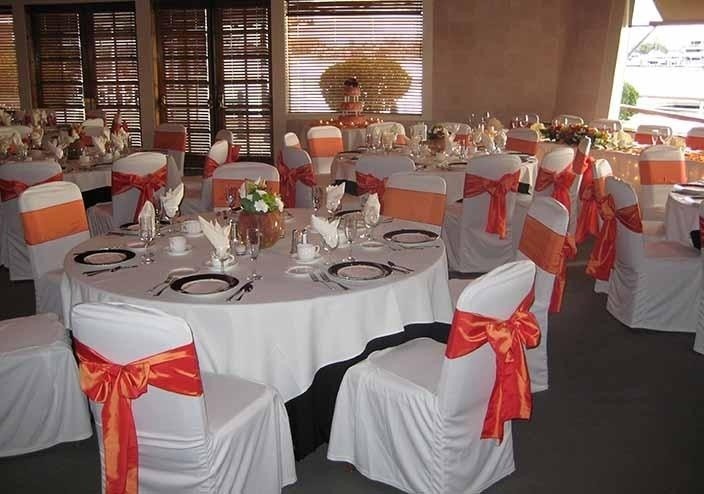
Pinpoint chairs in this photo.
[71,301,284,493]
[0,312,94,460]
[17,180,91,323]
[344,258,536,494]
[212,162,281,212]
[280,119,592,272]
[448,194,572,394]
[383,173,448,227]
[1,116,187,279]
[493,117,703,239]
[591,144,703,356]
[179,129,240,214]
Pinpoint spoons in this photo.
[380,260,416,275]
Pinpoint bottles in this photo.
[292,229,306,252]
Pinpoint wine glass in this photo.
[204,182,293,273]
[162,202,179,233]
[335,113,679,173]
[138,214,157,265]
[311,186,379,261]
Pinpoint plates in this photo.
[73,248,137,266]
[167,265,199,278]
[328,260,393,286]
[294,256,324,265]
[119,221,159,233]
[359,240,383,249]
[128,242,155,250]
[178,231,213,238]
[169,273,239,298]
[383,229,439,247]
[285,265,312,277]
[161,244,196,257]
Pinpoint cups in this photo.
[0,105,130,172]
[181,219,204,234]
[296,244,320,260]
[168,236,187,254]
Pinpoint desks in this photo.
[61,208,455,444]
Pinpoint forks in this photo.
[143,278,178,297]
[308,271,351,292]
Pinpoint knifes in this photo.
[226,280,254,303]
[79,265,138,275]
[88,266,122,277]
